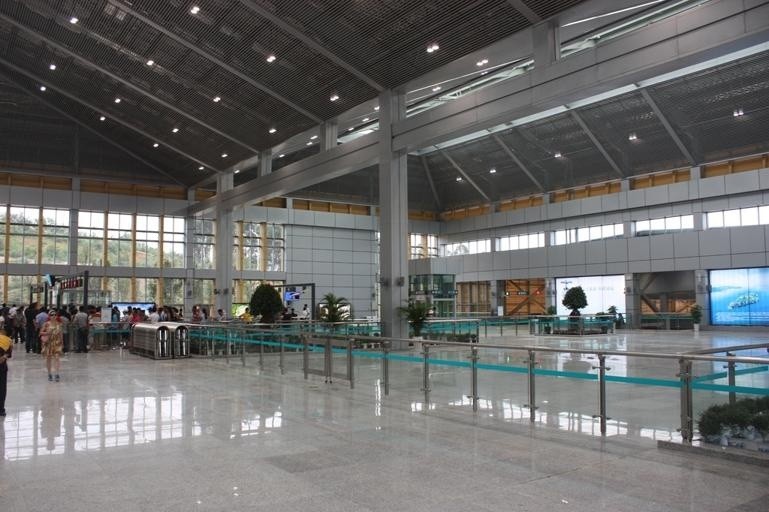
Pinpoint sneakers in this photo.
[48,374,52,381]
[55,374,59,382]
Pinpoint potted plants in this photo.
[695,394,768,447]
[691,304,703,332]
[397,295,434,353]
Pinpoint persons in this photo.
[39,310,64,382]
[0,329,13,416]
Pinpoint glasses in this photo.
[51,314,56,316]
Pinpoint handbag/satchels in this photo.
[40,334,50,343]
